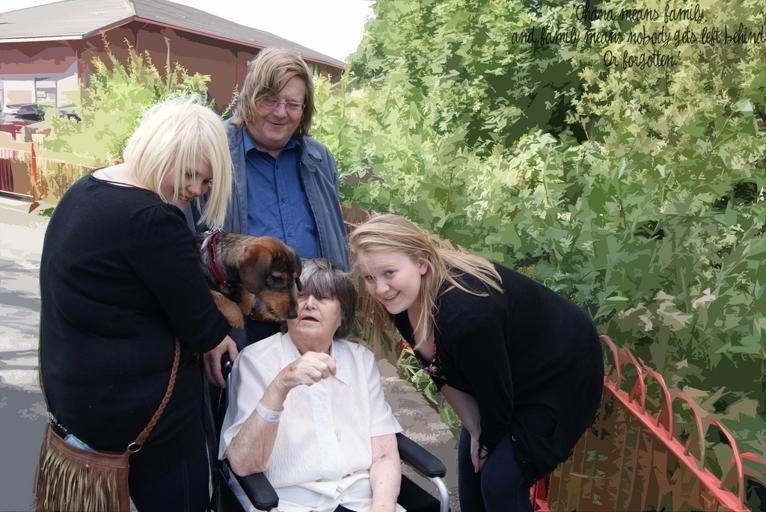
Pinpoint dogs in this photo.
[191,227,303,331]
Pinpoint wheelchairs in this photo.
[217,353,453,511]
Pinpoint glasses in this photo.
[265,98,307,116]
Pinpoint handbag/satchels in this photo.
[34,423,130,512]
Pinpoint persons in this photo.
[340,213,606,511]
[181,44,351,436]
[215,256,411,511]
[36,90,235,511]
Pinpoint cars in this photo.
[1,104,82,125]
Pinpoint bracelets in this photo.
[253,401,285,423]
